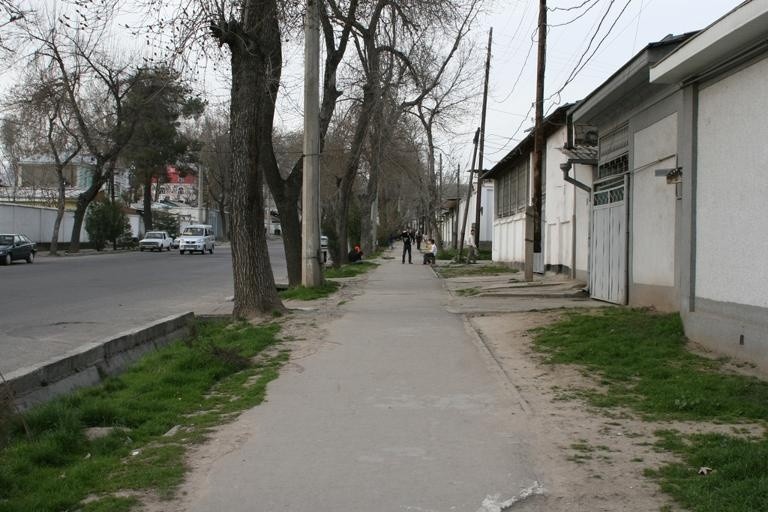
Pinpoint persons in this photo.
[400,226,438,265]
[349,247,361,262]
[466,230,478,264]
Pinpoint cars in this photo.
[138,230,180,252]
[0,233,38,266]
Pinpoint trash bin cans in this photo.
[320,236,328,271]
[275,230,280,235]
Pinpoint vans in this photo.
[180,224,215,254]
